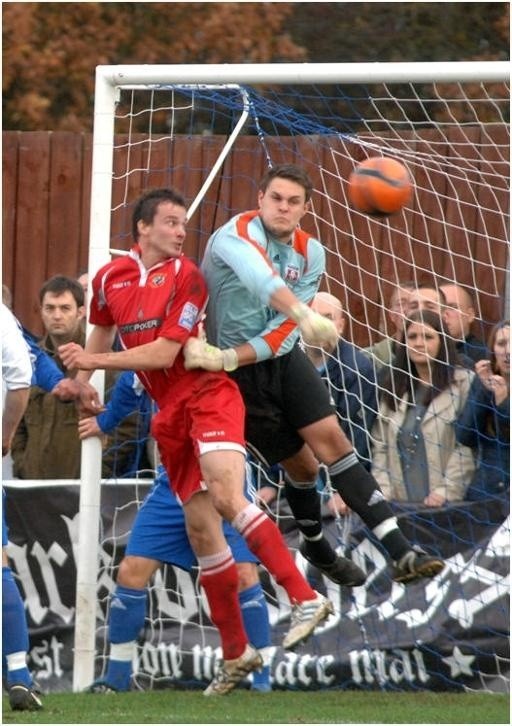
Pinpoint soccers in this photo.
[350,157,412,217]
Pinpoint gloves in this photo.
[287,303,337,346]
[184,336,238,372]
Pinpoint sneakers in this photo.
[282,590,335,650]
[299,539,367,587]
[202,643,263,696]
[84,681,119,694]
[390,544,445,584]
[7,682,43,710]
